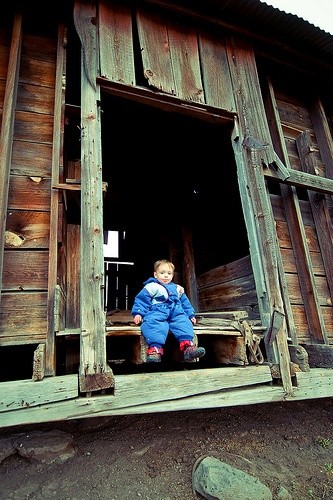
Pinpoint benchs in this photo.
[105,326,268,366]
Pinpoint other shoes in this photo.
[146,346,164,364]
[184,347,206,363]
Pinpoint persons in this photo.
[131,259,205,363]
